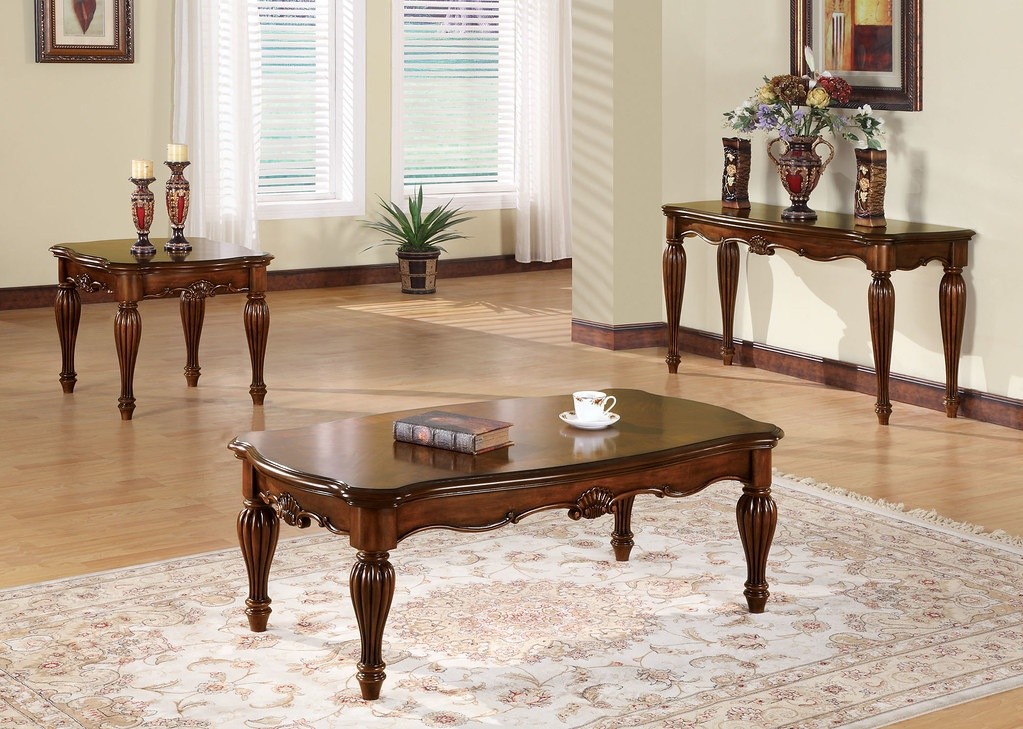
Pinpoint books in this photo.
[392,410,515,454]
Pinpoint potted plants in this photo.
[362,184,477,296]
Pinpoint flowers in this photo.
[722,46,887,152]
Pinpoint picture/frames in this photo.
[790,0,923,112]
[34,0,134,63]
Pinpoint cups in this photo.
[573,390,616,421]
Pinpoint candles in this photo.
[131,160,154,180]
[166,144,188,162]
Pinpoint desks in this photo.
[226,388,785,700]
[49,236,276,420]
[661,201,977,425]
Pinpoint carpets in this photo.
[1,470,1023,729]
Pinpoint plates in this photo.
[559,410,620,430]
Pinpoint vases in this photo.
[767,135,836,219]
[854,148,887,227]
[721,137,751,208]
